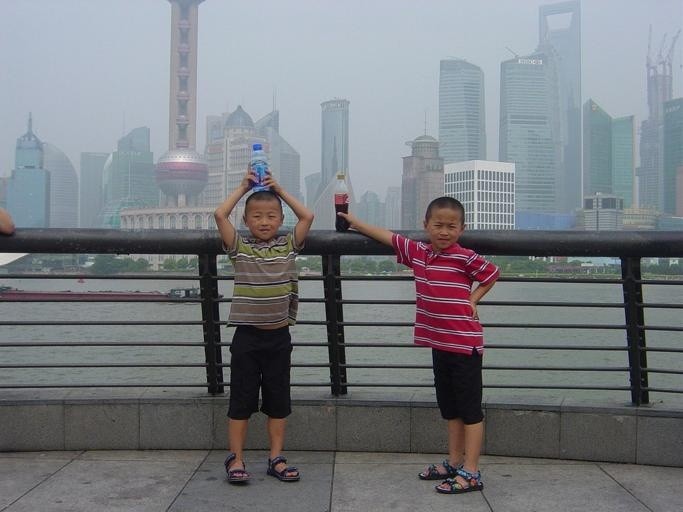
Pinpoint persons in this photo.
[0,206,15,234]
[337,197,500,494]
[214,162,315,483]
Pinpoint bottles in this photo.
[333,173,350,232]
[249,144,271,193]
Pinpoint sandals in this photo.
[418,459,464,480]
[224,453,250,482]
[435,469,482,494]
[267,455,301,482]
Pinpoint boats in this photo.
[0,284,225,303]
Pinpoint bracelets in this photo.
[280,191,286,197]
